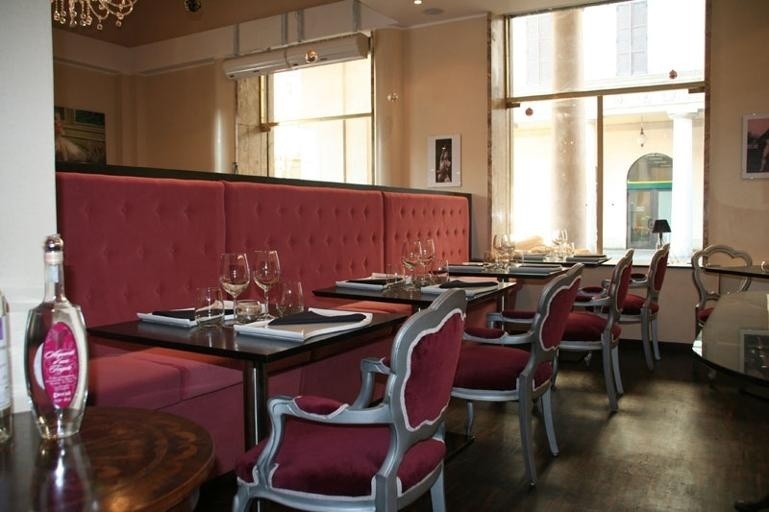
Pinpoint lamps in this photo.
[651,219,671,249]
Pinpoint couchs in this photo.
[55,160,473,481]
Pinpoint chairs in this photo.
[376,261,584,487]
[690,242,753,338]
[552,249,636,412]
[235,286,468,511]
[602,241,671,371]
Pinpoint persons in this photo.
[754,127,769,172]
[436,145,451,182]
[55,108,87,162]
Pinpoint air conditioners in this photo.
[220,33,369,82]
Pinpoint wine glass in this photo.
[217,251,251,330]
[417,239,436,285]
[251,250,277,321]
[399,240,422,292]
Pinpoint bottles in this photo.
[428,258,450,286]
[385,263,406,295]
[276,280,305,319]
[193,287,225,330]
[22,233,91,443]
[28,435,99,512]
[0,288,15,446]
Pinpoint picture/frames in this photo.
[737,328,769,380]
[427,133,462,188]
[738,111,769,182]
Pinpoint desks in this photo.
[312,270,518,327]
[1,407,215,511]
[429,257,570,330]
[509,252,610,268]
[706,263,768,398]
[86,299,410,512]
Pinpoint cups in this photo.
[235,298,263,326]
[545,227,577,260]
[761,260,769,273]
[483,232,524,272]
